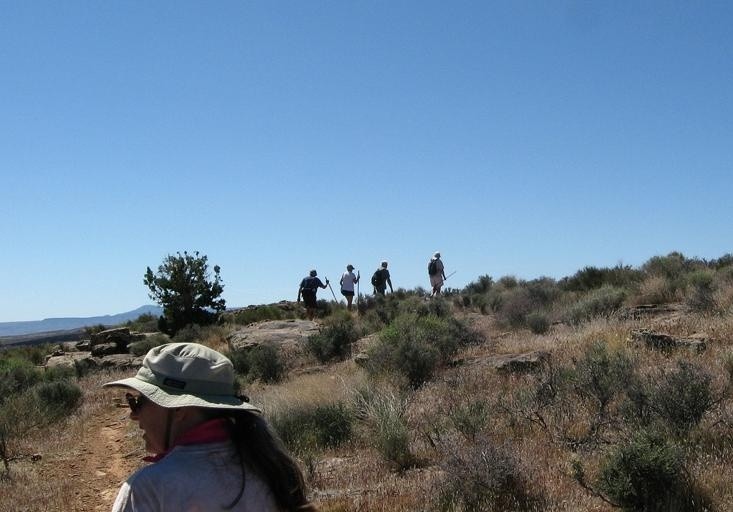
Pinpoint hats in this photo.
[435,253,440,257]
[382,262,387,267]
[347,265,355,269]
[102,342,262,412]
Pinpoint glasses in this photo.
[126,393,148,413]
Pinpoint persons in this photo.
[339,264,360,311]
[370,261,393,297]
[98,340,318,512]
[428,251,446,297]
[297,269,328,320]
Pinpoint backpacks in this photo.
[372,270,384,286]
[429,259,438,274]
